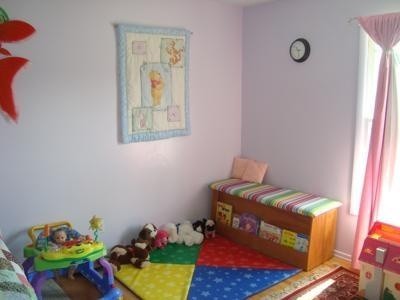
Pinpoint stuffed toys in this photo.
[111,218,216,269]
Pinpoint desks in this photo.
[211,177,338,272]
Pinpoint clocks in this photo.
[289,38,310,62]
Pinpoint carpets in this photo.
[281,265,369,300]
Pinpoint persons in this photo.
[53,229,79,280]
